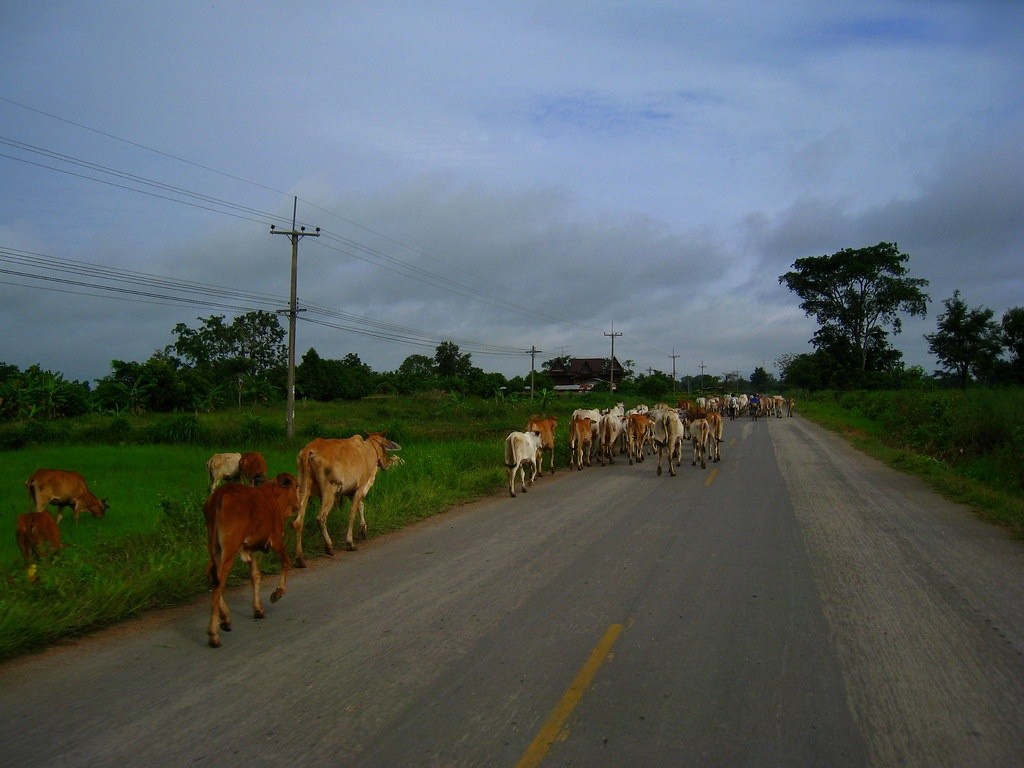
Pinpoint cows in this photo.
[200,426,402,649]
[24,467,112,527]
[14,510,71,569]
[503,392,795,498]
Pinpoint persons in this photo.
[776,399,782,418]
[705,392,715,409]
[787,399,794,417]
[750,394,760,421]
[729,394,736,421]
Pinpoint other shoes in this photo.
[730,418,732,421]
[755,419,757,421]
